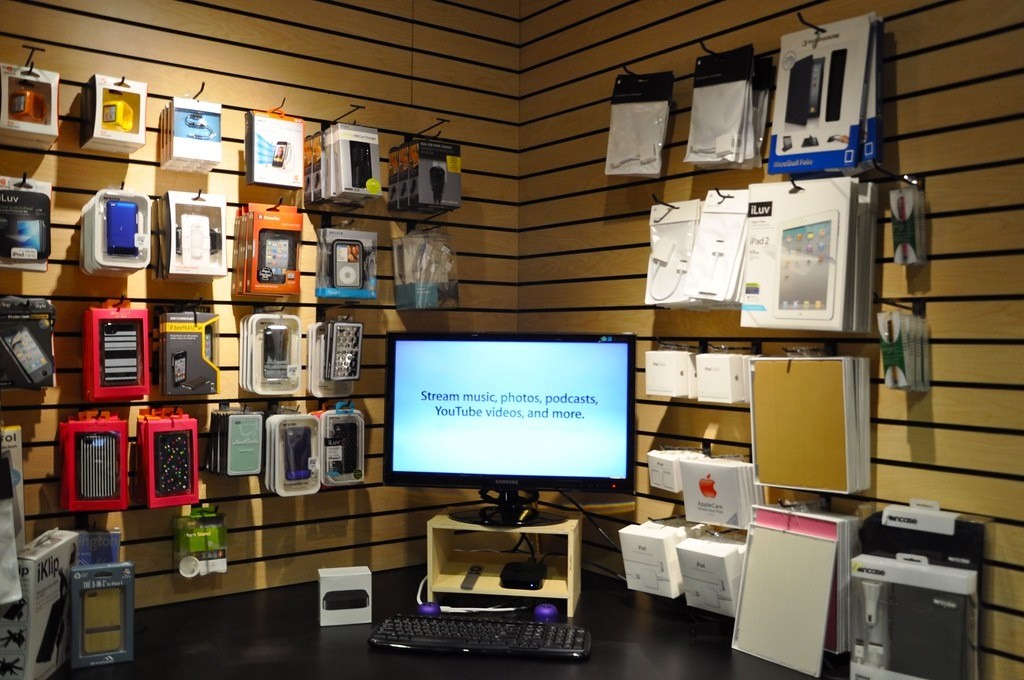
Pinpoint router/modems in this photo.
[501,561,543,590]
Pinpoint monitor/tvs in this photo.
[382,330,637,495]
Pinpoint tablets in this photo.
[781,48,847,152]
[774,209,840,319]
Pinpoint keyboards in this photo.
[369,611,593,662]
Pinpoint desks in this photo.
[58,553,853,680]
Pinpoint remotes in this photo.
[460,564,484,590]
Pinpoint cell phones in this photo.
[265,234,292,283]
[272,141,287,167]
[36,593,67,662]
[0,323,53,384]
[171,351,187,387]
